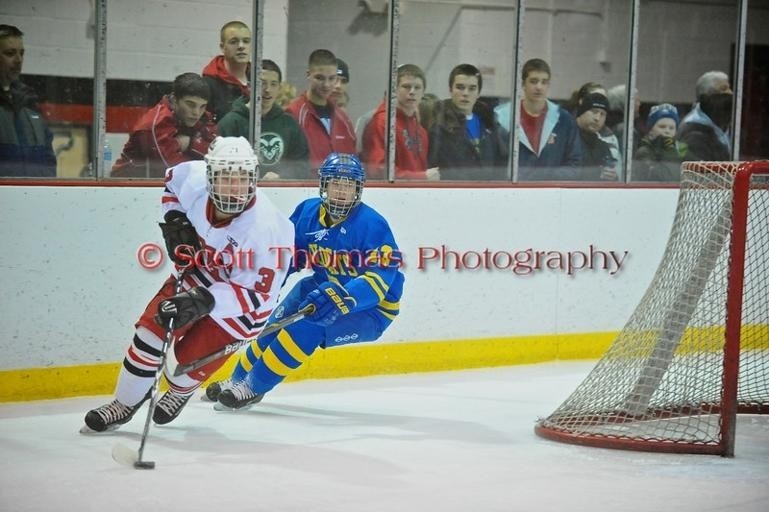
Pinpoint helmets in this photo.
[318,153,366,216]
[205,136,260,214]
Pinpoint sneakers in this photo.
[153,389,194,424]
[206,378,240,402]
[218,381,265,407]
[84,400,137,431]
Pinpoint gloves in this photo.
[154,287,214,329]
[299,282,348,325]
[159,218,201,264]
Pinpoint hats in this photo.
[579,94,610,117]
[646,104,679,133]
[335,58,349,82]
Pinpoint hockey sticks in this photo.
[113,267,187,469]
[166,305,316,376]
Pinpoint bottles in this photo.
[103,138,112,179]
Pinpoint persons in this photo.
[495,58,581,180]
[634,104,700,181]
[288,49,356,179]
[678,71,733,161]
[356,87,385,164]
[220,59,311,180]
[331,56,349,100]
[609,85,641,156]
[336,94,349,112]
[202,21,252,123]
[363,65,441,181]
[1,24,58,177]
[81,136,295,434]
[577,82,606,108]
[111,72,219,178]
[576,94,619,181]
[202,152,406,412]
[427,62,509,181]
